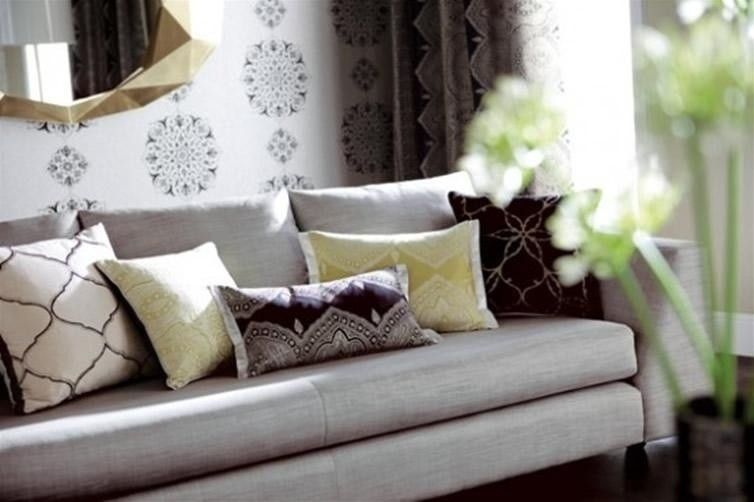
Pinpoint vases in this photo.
[674,393,754,502]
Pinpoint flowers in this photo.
[466,2,754,407]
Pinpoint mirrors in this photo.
[0,2,222,126]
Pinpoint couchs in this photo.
[0,163,715,502]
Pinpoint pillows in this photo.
[206,264,439,380]
[91,239,239,392]
[3,222,146,411]
[297,219,498,333]
[448,192,603,320]
